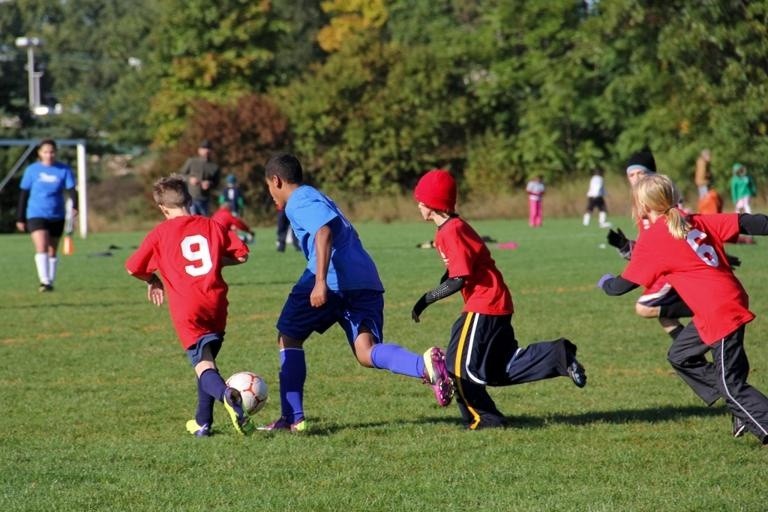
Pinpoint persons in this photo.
[598,174,768,447]
[526,174,545,228]
[256,154,455,434]
[583,165,611,228]
[181,140,302,254]
[413,168,585,431]
[691,150,755,245]
[127,174,256,439]
[16,140,77,291]
[605,148,742,341]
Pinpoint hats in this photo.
[413,171,457,216]
[625,149,656,174]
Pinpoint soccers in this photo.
[225,371,267,416]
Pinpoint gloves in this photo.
[607,227,628,249]
[599,273,614,286]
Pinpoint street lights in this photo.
[13,34,45,112]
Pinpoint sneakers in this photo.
[419,346,453,407]
[223,388,257,437]
[562,339,587,388]
[256,417,308,434]
[186,419,210,437]
[732,416,745,438]
